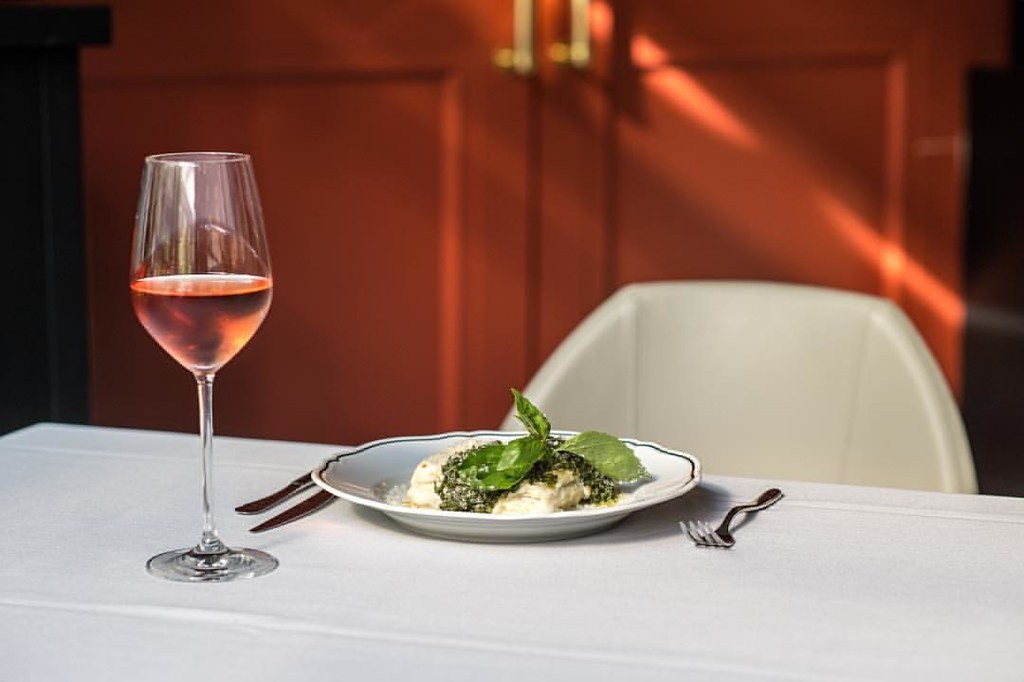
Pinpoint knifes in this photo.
[249,488,337,533]
[234,471,312,515]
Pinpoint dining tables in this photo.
[0,424,1024,682]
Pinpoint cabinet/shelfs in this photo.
[72,2,1001,444]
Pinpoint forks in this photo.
[678,487,783,549]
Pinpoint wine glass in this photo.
[129,150,279,582]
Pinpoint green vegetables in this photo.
[453,387,656,493]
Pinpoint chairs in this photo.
[514,281,977,492]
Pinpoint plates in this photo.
[313,428,702,540]
